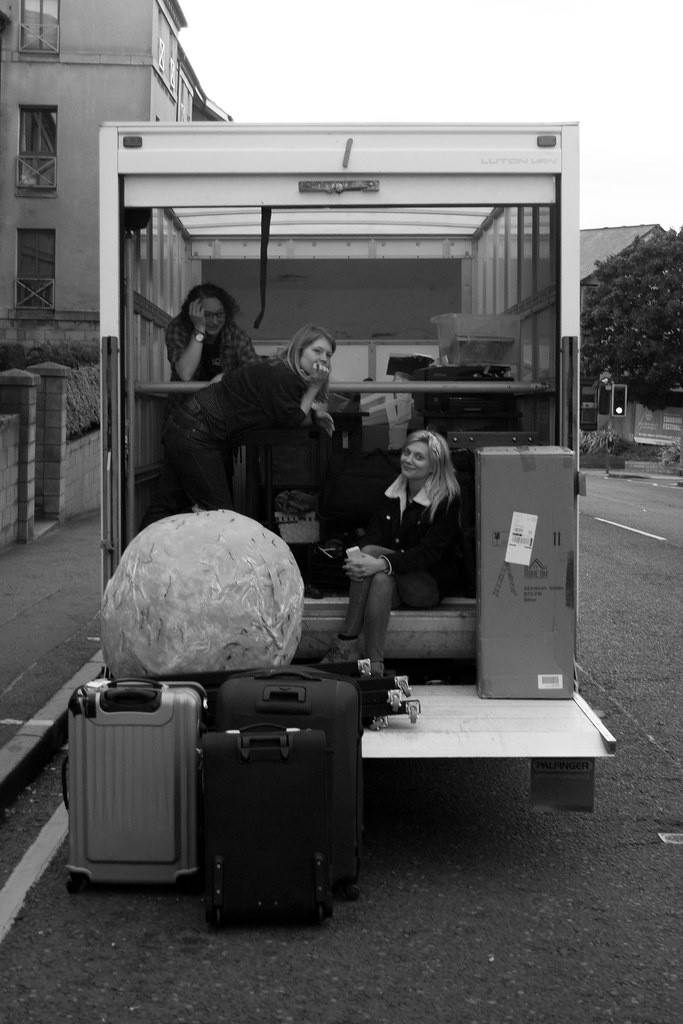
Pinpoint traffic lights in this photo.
[612,383,627,417]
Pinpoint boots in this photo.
[369,661,383,677]
[315,637,361,665]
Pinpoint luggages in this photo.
[197,722,335,932]
[61,675,208,897]
[215,665,365,901]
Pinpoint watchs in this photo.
[191,330,206,343]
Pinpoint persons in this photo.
[312,430,477,680]
[157,321,336,512]
[161,282,263,388]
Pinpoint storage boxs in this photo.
[274,511,319,543]
[437,313,520,367]
[475,446,575,699]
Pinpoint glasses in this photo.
[204,311,227,319]
